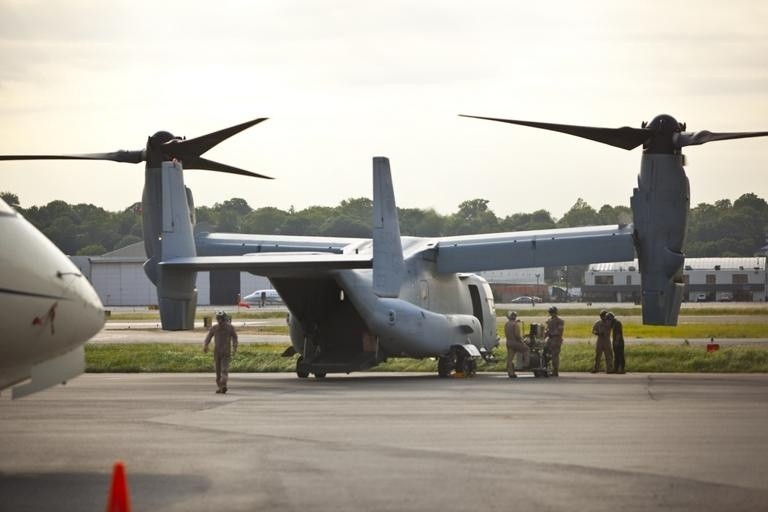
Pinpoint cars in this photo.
[511,296,543,303]
[696,294,706,301]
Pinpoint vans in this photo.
[720,293,729,302]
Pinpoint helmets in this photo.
[549,307,557,315]
[215,311,225,320]
[506,311,517,319]
[600,310,614,321]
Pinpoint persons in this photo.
[544,305,564,377]
[202,311,239,394]
[591,309,616,375]
[605,311,626,374]
[504,310,529,378]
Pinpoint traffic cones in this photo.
[108,459,134,511]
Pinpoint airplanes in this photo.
[243,288,283,307]
[0,194,112,403]
[1,110,767,382]
